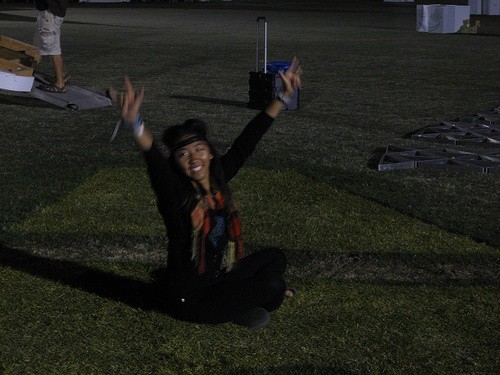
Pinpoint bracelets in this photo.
[276,92,290,103]
[126,113,144,138]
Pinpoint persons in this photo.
[106,55,303,328]
[32,0,66,92]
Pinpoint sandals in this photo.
[44,82,66,93]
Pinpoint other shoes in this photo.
[230,302,271,327]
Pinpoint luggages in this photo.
[247,15,300,111]
